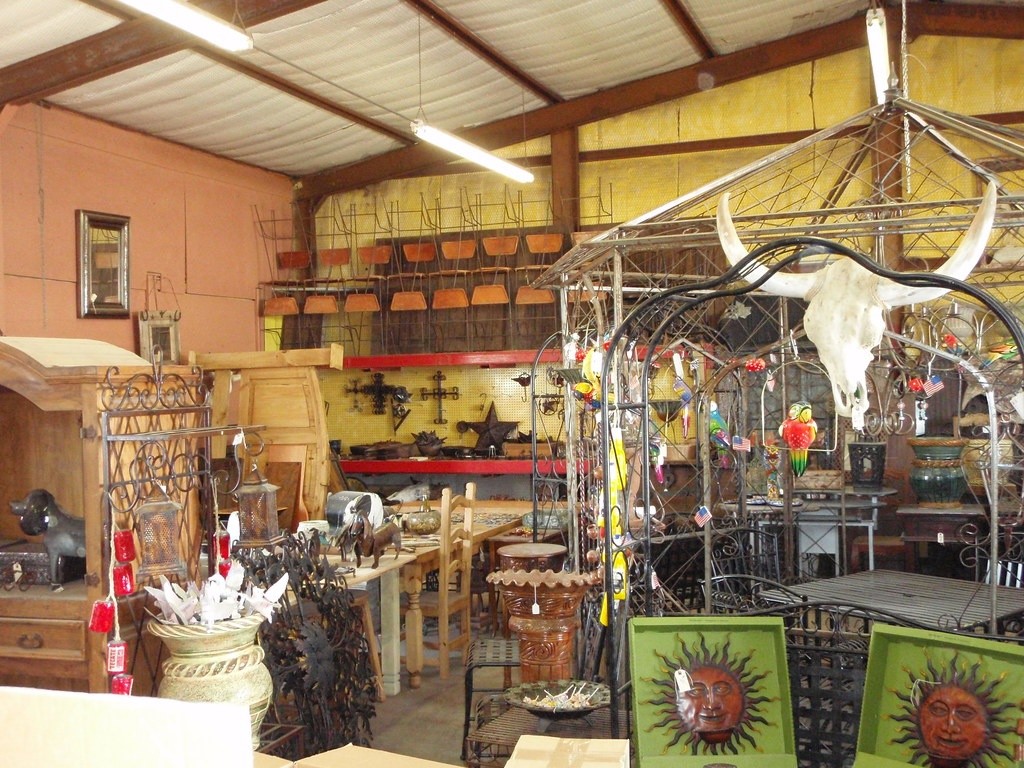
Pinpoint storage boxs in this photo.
[502,442,557,456]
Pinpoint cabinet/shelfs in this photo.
[718,488,899,582]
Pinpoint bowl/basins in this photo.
[350,445,373,455]
[442,446,471,455]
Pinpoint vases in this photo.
[847,442,888,490]
[147,612,272,751]
[907,437,969,509]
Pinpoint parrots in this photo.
[709,400,731,469]
[649,433,664,484]
[673,374,691,439]
[779,401,817,479]
[574,383,615,409]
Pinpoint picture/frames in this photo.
[138,310,182,365]
[75,209,131,318]
[974,156,1024,268]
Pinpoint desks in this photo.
[319,505,522,690]
[339,456,589,475]
[273,554,417,697]
[896,503,985,580]
[465,706,633,747]
[757,568,1024,646]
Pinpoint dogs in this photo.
[9,489,85,591]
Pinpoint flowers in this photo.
[779,418,817,475]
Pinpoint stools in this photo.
[490,528,559,632]
[851,536,904,573]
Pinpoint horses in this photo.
[350,510,401,568]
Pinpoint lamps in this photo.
[866,0,890,114]
[117,0,254,53]
[410,0,534,183]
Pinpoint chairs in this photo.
[253,176,612,354]
[400,482,476,679]
[712,529,781,612]
[460,640,521,761]
